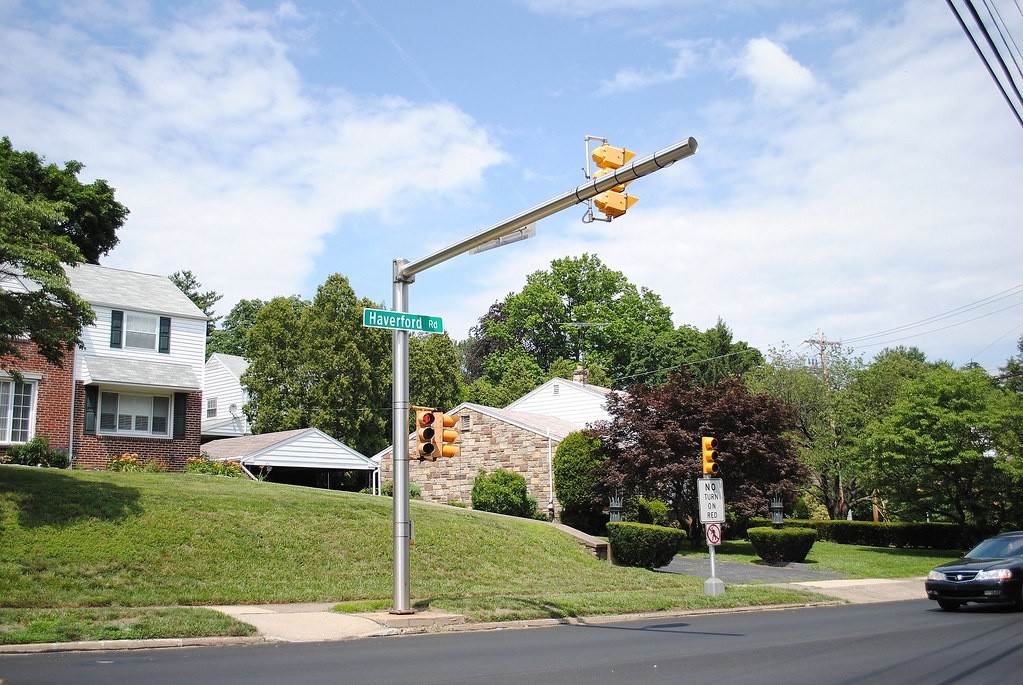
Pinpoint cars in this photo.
[925,531,1023,610]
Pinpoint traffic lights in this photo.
[702,437,719,475]
[415,410,438,460]
[436,411,460,458]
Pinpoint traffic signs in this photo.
[363,307,444,334]
